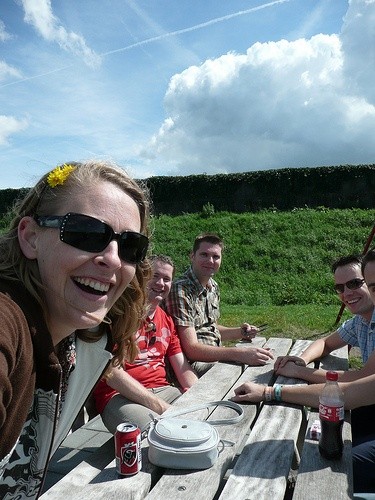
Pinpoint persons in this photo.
[231,245,375,493]
[94,254,200,435]
[0,161,153,500]
[161,234,274,377]
[274,253,375,384]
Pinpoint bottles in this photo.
[319,371,345,461]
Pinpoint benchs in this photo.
[38,412,113,500]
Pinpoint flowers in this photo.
[46,164,77,187]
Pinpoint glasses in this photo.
[34,212,150,263]
[148,322,156,346]
[335,279,365,293]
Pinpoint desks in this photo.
[76,337,353,500]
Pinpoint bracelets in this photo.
[265,387,271,402]
[274,385,282,401]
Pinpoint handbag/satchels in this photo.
[147,401,244,469]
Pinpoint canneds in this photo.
[114,422,143,476]
